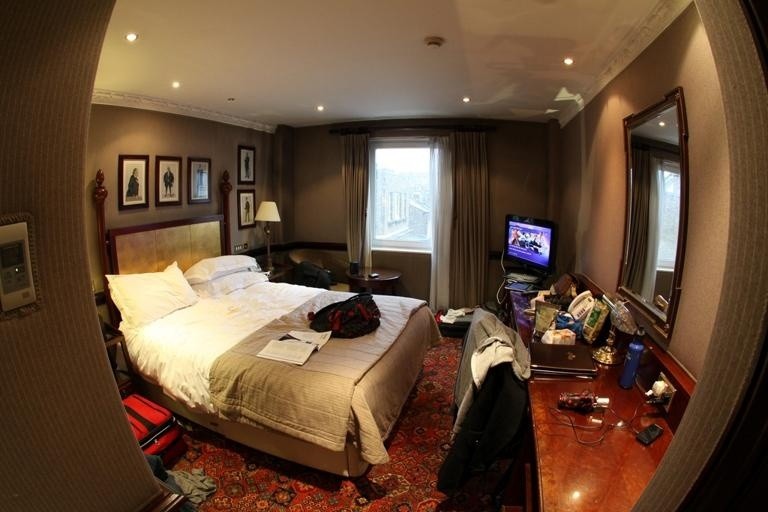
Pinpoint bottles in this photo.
[556,391,610,411]
[619,325,645,390]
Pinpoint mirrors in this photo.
[615,86,690,352]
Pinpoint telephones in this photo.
[567,290,595,322]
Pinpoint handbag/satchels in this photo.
[309,288,381,340]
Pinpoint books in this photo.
[256,330,333,366]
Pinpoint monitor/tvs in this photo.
[503,213,559,274]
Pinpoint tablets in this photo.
[504,282,533,292]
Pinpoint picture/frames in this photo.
[119,155,149,210]
[238,144,255,185]
[237,189,256,229]
[155,155,182,206]
[187,157,212,204]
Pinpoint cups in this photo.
[348,260,364,276]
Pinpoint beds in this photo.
[93,167,444,479]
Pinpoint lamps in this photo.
[254,201,282,275]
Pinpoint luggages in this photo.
[436,307,472,338]
[122,394,186,466]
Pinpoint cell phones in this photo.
[636,423,664,445]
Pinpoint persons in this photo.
[245,152,250,179]
[244,197,250,222]
[127,168,139,196]
[197,164,204,194]
[512,230,542,255]
[164,167,174,197]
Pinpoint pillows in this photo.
[183,254,261,285]
[104,261,199,330]
[190,271,269,302]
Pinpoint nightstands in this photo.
[260,263,295,284]
[100,321,150,400]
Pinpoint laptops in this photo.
[528,342,598,377]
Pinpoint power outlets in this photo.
[653,371,676,415]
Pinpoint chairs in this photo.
[451,307,536,511]
[288,247,352,292]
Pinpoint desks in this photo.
[345,266,403,295]
[507,275,673,512]
